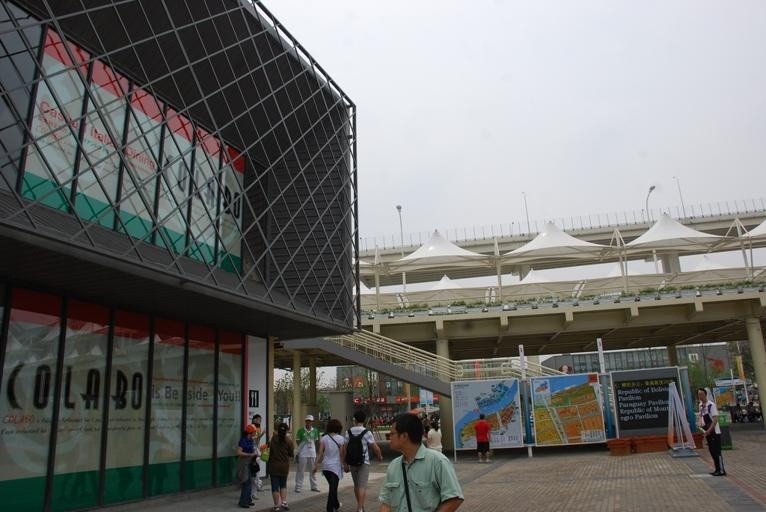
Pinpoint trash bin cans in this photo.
[718,414,732,449]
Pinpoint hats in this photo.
[244,424,261,434]
[303,415,315,422]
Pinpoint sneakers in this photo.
[477,458,492,463]
[238,487,366,512]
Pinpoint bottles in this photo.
[698,426,705,433]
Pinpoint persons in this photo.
[697,386,726,477]
[733,402,761,423]
[235,410,492,512]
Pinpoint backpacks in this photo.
[346,428,372,467]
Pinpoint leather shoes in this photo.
[709,471,727,476]
